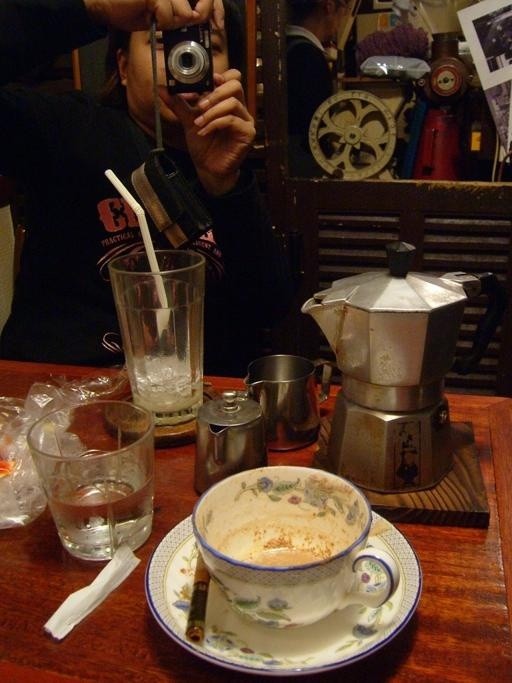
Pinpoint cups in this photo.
[104,250,209,429]
[30,400,156,562]
[192,462,404,632]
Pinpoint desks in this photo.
[0,361,512,683]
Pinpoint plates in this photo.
[145,507,421,677]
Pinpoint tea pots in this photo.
[241,354,335,453]
[300,238,502,492]
[190,389,266,496]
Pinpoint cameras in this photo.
[162,0,214,95]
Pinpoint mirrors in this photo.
[260,0,511,184]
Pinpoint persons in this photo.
[0,0,313,377]
[234,0,351,176]
